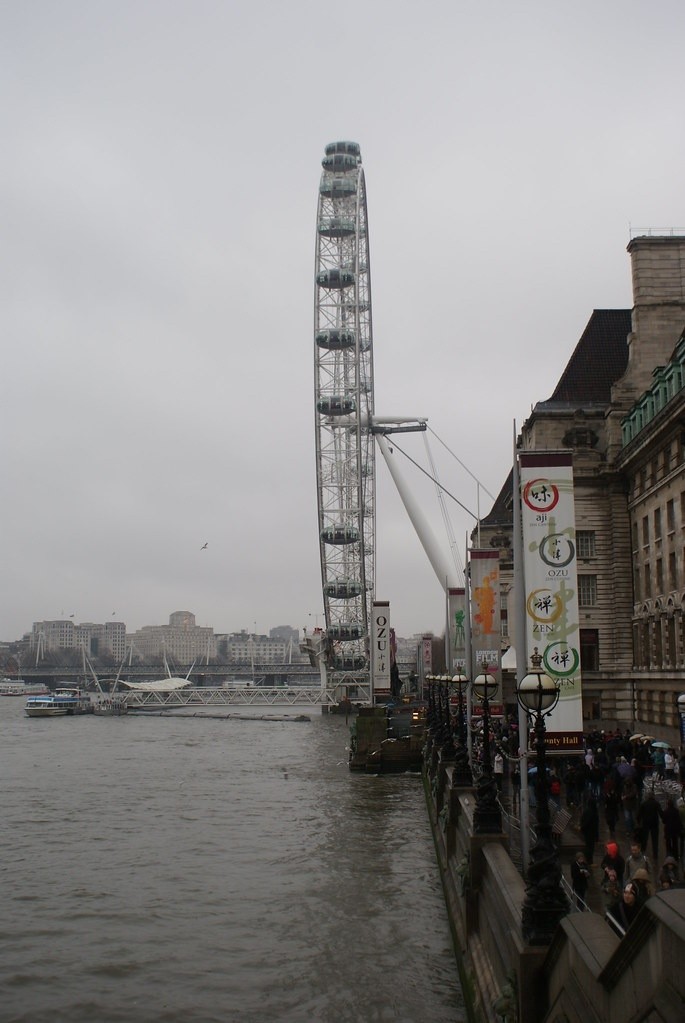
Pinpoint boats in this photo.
[93,699,129,716]
[0,678,50,695]
[24,687,91,716]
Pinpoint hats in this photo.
[610,870,617,877]
[624,884,637,895]
[576,852,584,860]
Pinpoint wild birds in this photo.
[201,542,208,550]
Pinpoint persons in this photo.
[98,696,123,709]
[449,704,685,940]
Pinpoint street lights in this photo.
[425,671,443,746]
[471,656,503,835]
[441,669,453,761]
[515,647,572,947]
[451,665,473,787]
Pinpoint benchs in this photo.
[545,810,573,844]
[531,799,558,821]
